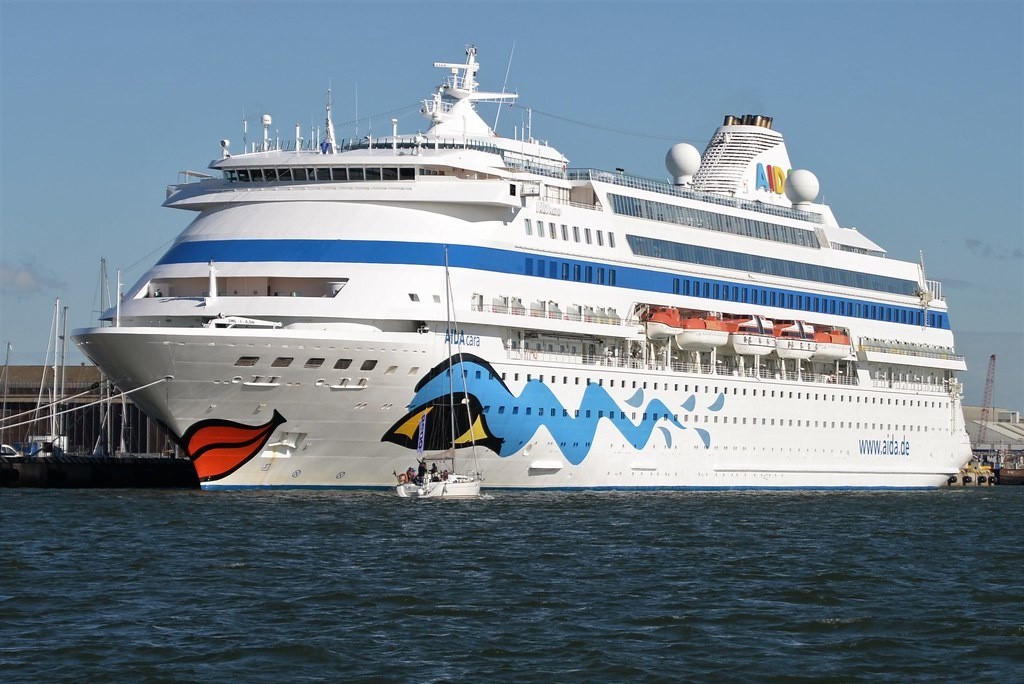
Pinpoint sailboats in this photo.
[393,244,487,501]
[0,257,181,458]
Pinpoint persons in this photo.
[829,370,836,384]
[154,289,163,297]
[393,457,448,485]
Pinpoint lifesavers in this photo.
[978,476,987,483]
[949,476,957,483]
[963,476,972,483]
[988,476,998,483]
[399,473,408,482]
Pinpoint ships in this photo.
[69,41,974,493]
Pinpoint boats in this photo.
[672,314,730,353]
[769,322,819,362]
[814,330,852,363]
[640,305,683,341]
[943,455,996,487]
[725,317,777,358]
[998,451,1024,484]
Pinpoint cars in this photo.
[1,444,20,458]
